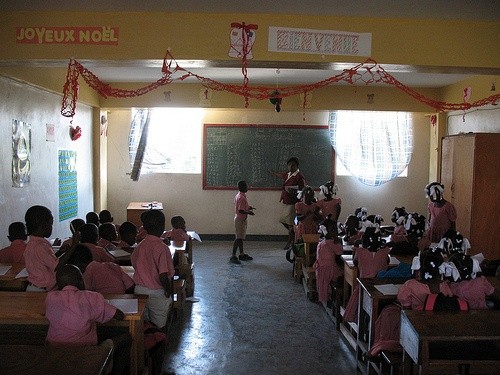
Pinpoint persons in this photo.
[398,252,454,311]
[390,206,408,234]
[314,214,342,304]
[163,216,200,302]
[441,251,495,310]
[343,235,391,324]
[318,182,341,221]
[230,180,256,265]
[130,210,176,375]
[45,263,131,352]
[292,185,321,242]
[70,210,147,263]
[70,244,135,294]
[419,181,457,249]
[275,157,304,250]
[23,205,80,292]
[344,207,384,244]
[0,222,28,262]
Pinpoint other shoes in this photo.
[238,254,252,260]
[230,257,241,264]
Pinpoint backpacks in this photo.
[423,294,469,312]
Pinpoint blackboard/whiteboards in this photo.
[202,124,335,190]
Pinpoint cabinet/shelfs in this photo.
[439,133,500,260]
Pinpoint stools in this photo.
[379,347,414,375]
[330,281,343,331]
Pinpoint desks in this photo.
[0,201,198,375]
[304,226,500,375]
